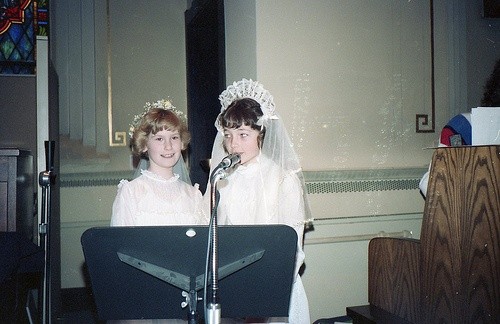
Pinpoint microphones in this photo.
[219,163,240,179]
[219,153,240,169]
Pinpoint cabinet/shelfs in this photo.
[0,147,34,239]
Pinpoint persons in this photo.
[109,98,208,226]
[203,78,316,324]
